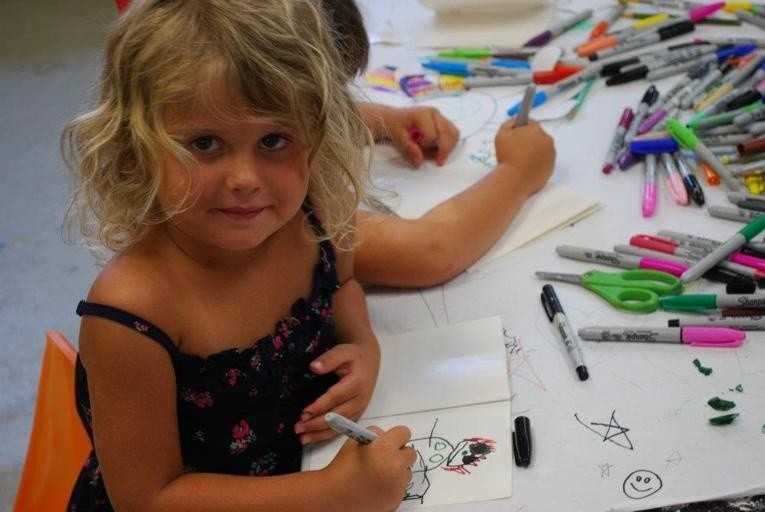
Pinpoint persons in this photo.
[297,0,557,289]
[59,0,418,511]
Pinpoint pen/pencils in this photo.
[601,0,765,220]
[511,81,536,129]
[556,232,765,332]
[571,325,746,347]
[324,412,379,444]
[419,7,594,91]
[507,61,603,115]
[539,284,592,384]
[678,211,765,284]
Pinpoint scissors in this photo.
[535,269,682,313]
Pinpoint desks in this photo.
[299,0,765,512]
